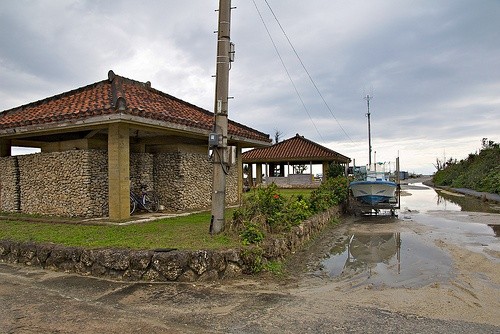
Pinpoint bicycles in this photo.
[129,176,160,215]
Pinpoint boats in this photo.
[348,171,396,206]
